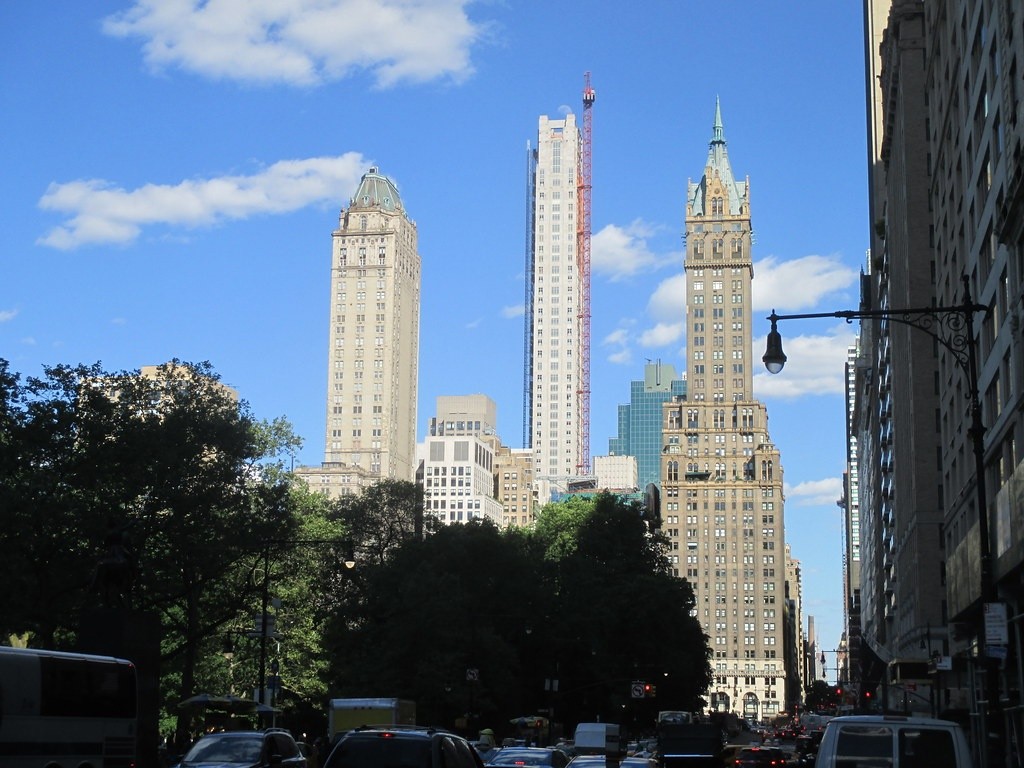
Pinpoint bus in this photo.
[0,647,138,768]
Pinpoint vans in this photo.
[811,715,975,768]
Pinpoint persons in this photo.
[473,728,497,761]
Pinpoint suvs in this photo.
[171,727,307,768]
[322,725,486,768]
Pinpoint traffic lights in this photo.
[651,684,657,698]
[644,684,651,692]
[866,687,875,698]
[835,686,843,695]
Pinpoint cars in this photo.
[467,715,824,767]
[297,741,317,768]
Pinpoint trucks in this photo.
[329,697,417,752]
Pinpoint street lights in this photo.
[821,667,849,685]
[762,273,1008,768]
[919,621,936,716]
[819,649,859,713]
